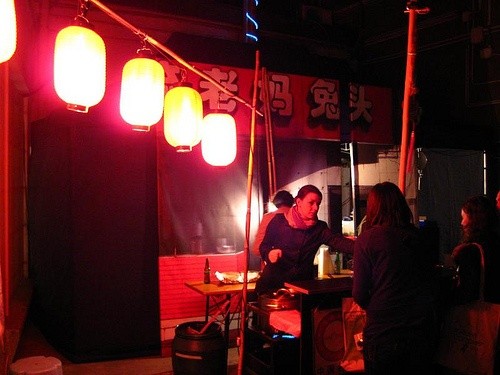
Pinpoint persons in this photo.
[352,181,441,375]
[451,197,500,300]
[254,190,294,255]
[252,185,355,375]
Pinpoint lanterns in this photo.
[0,0,16,63]
[54,26,107,113]
[201,109,237,167]
[164,82,203,152]
[120,52,164,132]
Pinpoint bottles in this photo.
[204,258,211,284]
[334,252,341,274]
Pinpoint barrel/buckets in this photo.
[170,320,228,375]
[9,357,63,375]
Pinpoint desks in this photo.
[186,280,256,363]
[284,277,353,375]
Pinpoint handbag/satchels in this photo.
[337,298,368,372]
[431,241,500,375]
